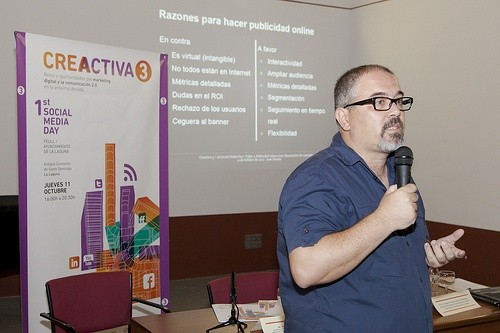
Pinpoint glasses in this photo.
[344,96,413,111]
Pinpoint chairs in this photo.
[206,272,281,309]
[40,270,171,332]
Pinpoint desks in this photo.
[127,277,500,333]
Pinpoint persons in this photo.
[276,64,466,333]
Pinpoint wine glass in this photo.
[439,270,456,294]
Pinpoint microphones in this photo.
[395,146,414,189]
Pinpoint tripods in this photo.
[206,272,248,333]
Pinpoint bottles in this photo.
[428,266,440,298]
